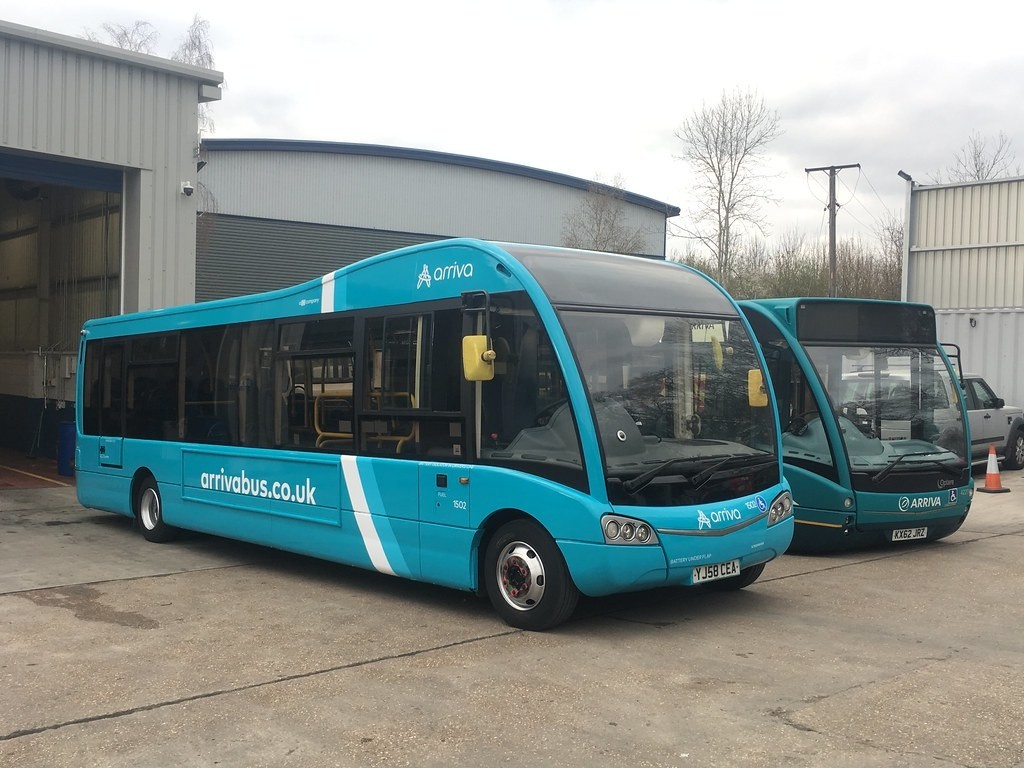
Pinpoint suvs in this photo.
[835,370,1024,473]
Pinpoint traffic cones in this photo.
[974,443,1011,492]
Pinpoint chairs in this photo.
[287,385,309,423]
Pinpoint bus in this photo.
[74,238,797,630]
[621,298,976,551]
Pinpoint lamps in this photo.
[197,161,207,173]
